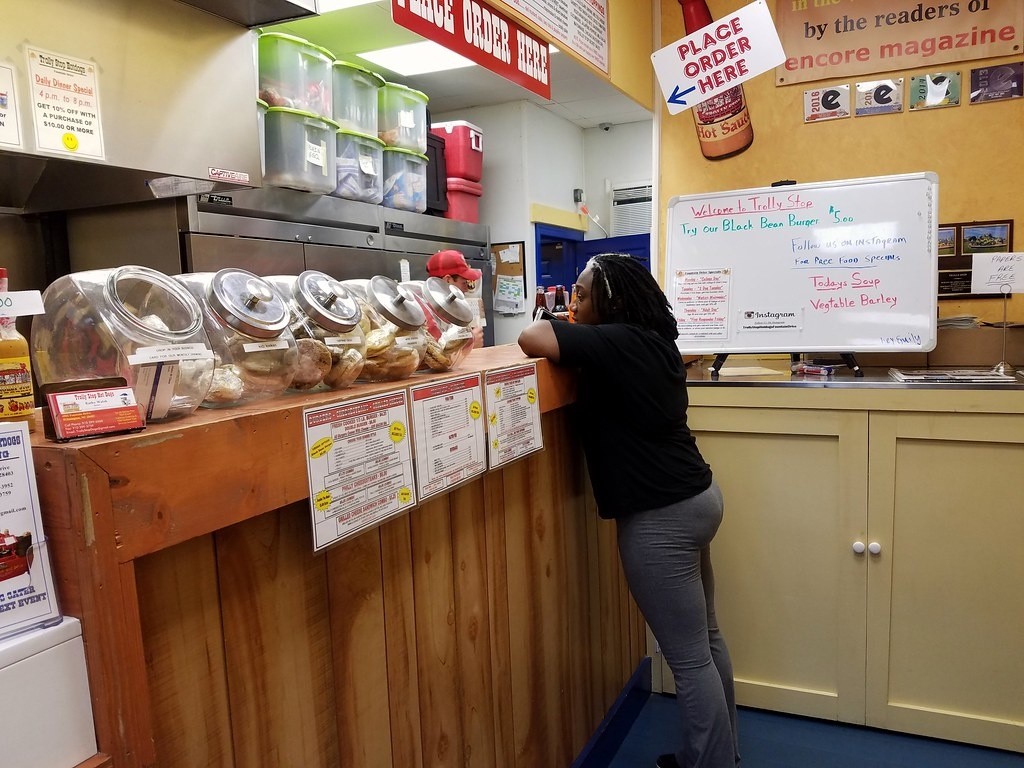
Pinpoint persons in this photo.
[517,254,743,768]
[425,250,485,349]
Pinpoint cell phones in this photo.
[533,307,559,322]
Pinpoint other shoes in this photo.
[656,752,681,768]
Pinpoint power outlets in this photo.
[574,189,583,203]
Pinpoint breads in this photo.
[140,297,466,404]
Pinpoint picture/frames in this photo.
[938,219,1013,300]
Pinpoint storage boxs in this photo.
[331,60,387,138]
[382,146,430,214]
[431,120,483,181]
[264,106,339,195]
[378,81,429,156]
[259,26,336,122]
[434,178,483,223]
[331,130,384,205]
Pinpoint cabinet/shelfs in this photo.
[651,387,1024,755]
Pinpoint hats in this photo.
[427,250,481,280]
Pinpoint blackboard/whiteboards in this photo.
[663,171,938,354]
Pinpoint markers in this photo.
[791,363,835,376]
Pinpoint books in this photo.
[889,368,1015,383]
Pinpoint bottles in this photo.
[532,286,550,322]
[31,264,474,423]
[0,268,36,433]
[676,1,754,161]
[568,284,578,324]
[552,285,570,323]
